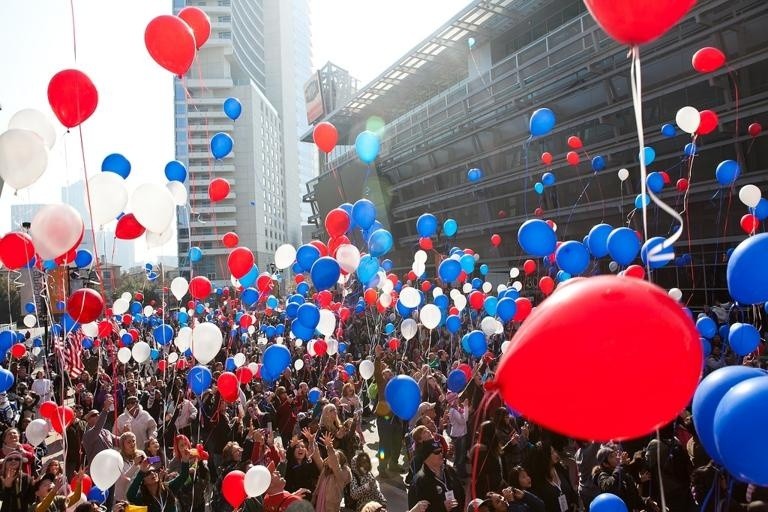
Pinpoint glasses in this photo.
[144,468,156,478]
[432,448,443,455]
[6,457,20,462]
[280,391,287,394]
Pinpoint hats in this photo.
[32,472,54,490]
[419,442,442,461]
[266,460,275,474]
[417,401,436,414]
[445,392,459,401]
[468,497,491,510]
[594,448,616,465]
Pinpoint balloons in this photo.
[2,2,768,512]
